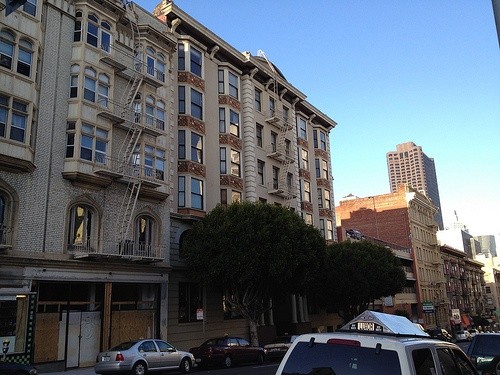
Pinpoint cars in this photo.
[0,360,38,375]
[265,334,300,360]
[93,338,196,375]
[455,329,473,342]
[426,328,453,342]
[187,336,266,370]
[466,328,479,339]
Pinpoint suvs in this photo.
[467,333,500,375]
[274,328,482,375]
